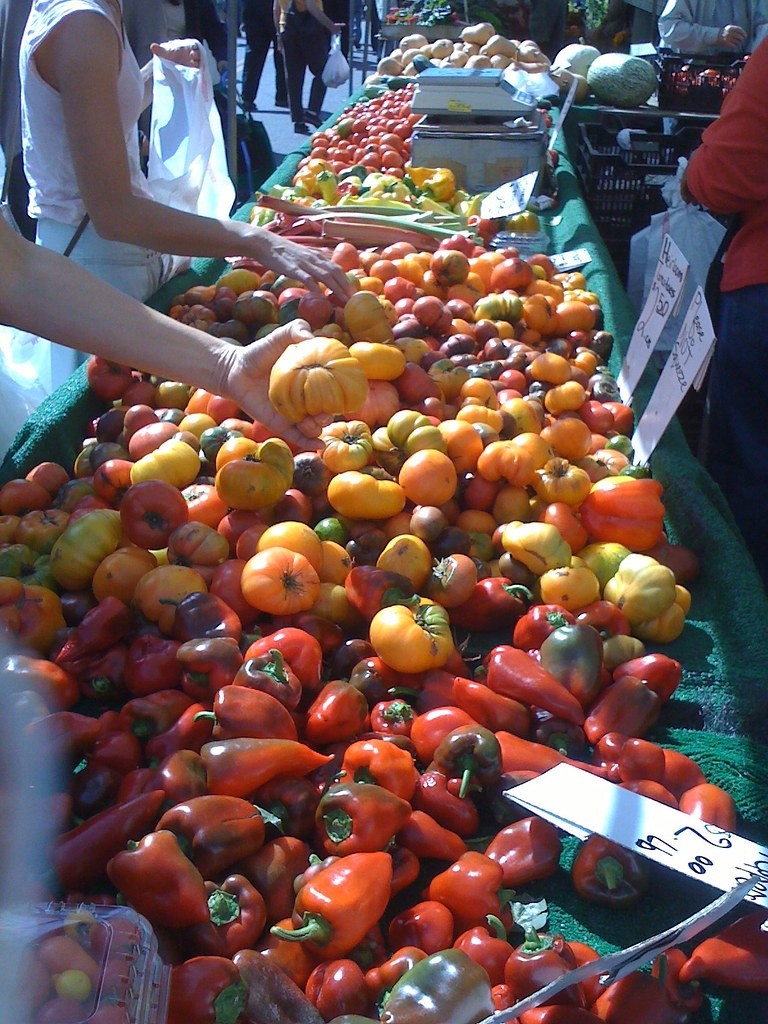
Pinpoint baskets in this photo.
[580,122,706,249]
[655,47,752,113]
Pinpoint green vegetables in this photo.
[394,0,454,27]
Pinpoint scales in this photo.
[411,67,539,116]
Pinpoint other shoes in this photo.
[241,102,257,111]
[294,111,323,133]
[275,101,289,108]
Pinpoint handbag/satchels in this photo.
[147,37,236,221]
[322,30,350,88]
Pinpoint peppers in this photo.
[0,563,768,1024]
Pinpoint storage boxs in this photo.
[653,47,752,113]
[574,121,707,290]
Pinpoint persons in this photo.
[272,0,346,133]
[20,0,361,393]
[119,0,164,177]
[160,0,228,142]
[214,0,242,38]
[238,0,289,112]
[0,0,38,242]
[682,32,768,585]
[487,0,568,61]
[0,211,334,451]
[658,0,768,57]
[322,0,415,64]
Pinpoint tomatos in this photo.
[668,69,736,100]
[0,237,700,690]
[293,82,426,184]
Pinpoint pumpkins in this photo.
[364,21,658,109]
